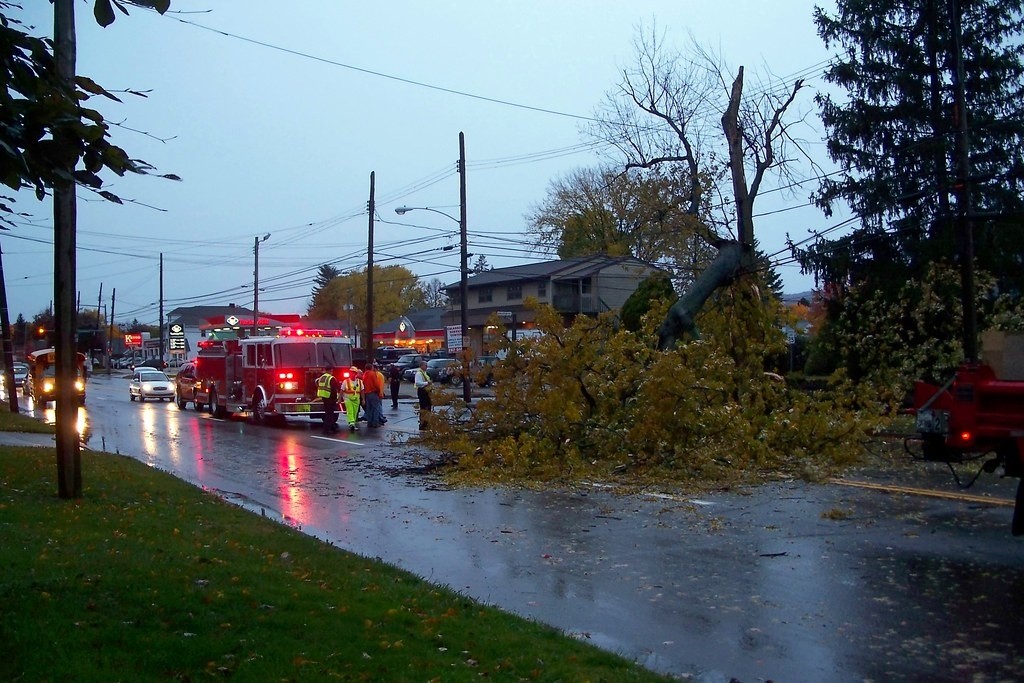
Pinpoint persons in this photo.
[414,361,434,431]
[387,363,402,410]
[340,366,364,433]
[315,365,340,435]
[359,357,389,428]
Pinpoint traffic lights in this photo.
[39,323,43,334]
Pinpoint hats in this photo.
[325,365,336,369]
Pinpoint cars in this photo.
[129,366,175,402]
[111,357,141,369]
[165,358,190,367]
[84,357,93,376]
[0,370,4,386]
[22,374,30,395]
[93,358,99,366]
[12,366,28,386]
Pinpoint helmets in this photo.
[349,366,357,373]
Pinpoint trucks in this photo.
[908,361,1024,542]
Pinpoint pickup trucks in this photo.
[422,350,456,362]
[382,354,439,382]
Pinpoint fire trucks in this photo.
[175,329,354,424]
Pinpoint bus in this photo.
[28,348,87,406]
[350,346,418,370]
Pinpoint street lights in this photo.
[253,233,271,336]
[395,207,471,404]
[118,324,125,369]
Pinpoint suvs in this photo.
[130,358,167,371]
[438,356,502,388]
[403,359,463,386]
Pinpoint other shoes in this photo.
[373,424,381,427]
[367,424,373,427]
[354,427,358,430]
[393,403,397,408]
[391,405,398,407]
[349,424,354,433]
